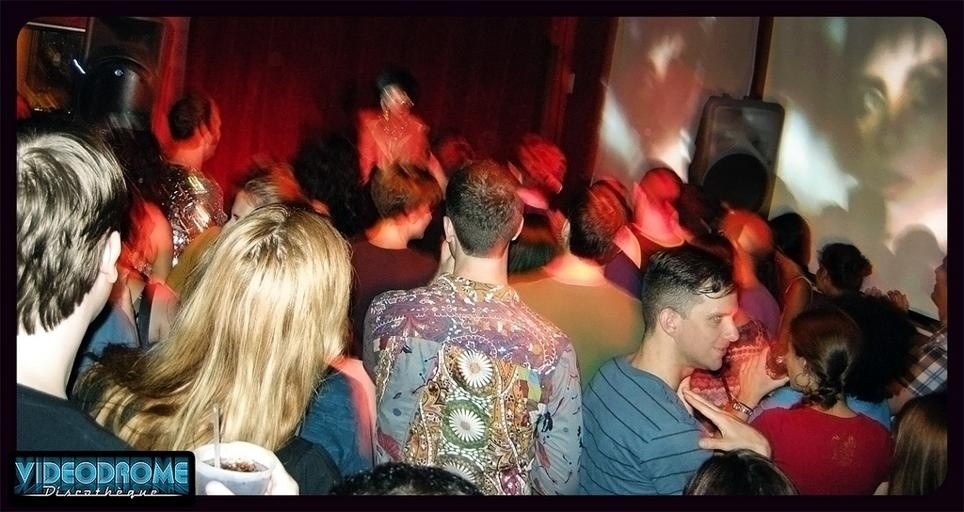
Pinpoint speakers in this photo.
[688,94,785,220]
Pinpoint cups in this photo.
[767,343,788,380]
[187,443,270,495]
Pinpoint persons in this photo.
[13,69,955,497]
